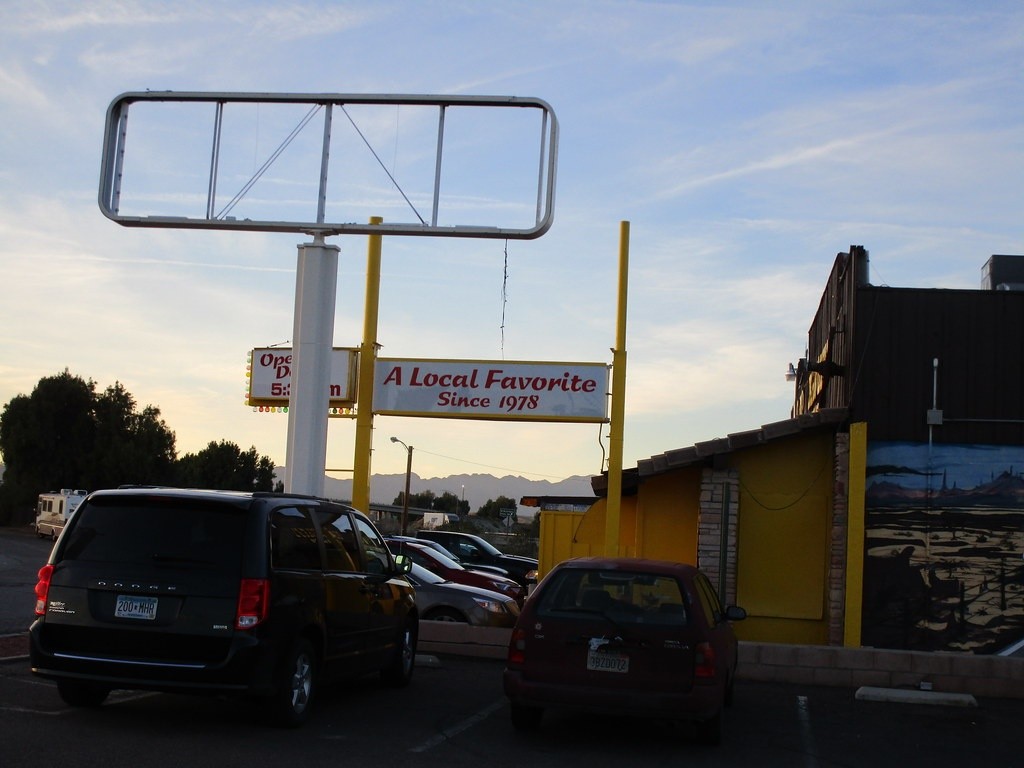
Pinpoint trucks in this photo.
[33,488,88,540]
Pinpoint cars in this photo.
[504,559,748,744]
[367,551,520,628]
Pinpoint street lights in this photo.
[391,437,413,535]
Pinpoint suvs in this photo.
[417,530,539,591]
[383,535,525,608]
[28,483,419,728]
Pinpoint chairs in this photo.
[584,590,611,612]
